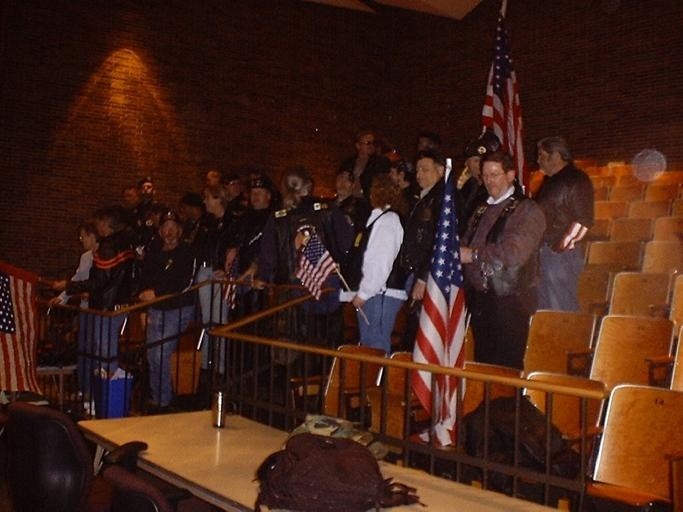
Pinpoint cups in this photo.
[211,391,229,428]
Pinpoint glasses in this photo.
[359,140,373,146]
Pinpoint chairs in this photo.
[0,401,194,512]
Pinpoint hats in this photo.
[159,210,178,226]
[463,140,492,159]
[137,175,154,186]
[247,178,271,189]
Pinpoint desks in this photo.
[77,408,571,512]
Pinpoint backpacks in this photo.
[251,432,427,512]
[280,413,389,461]
[457,394,583,494]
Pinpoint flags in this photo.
[0,262,45,399]
[478,4,532,198]
[407,159,470,451]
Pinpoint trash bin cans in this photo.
[92,375,134,420]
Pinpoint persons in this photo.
[43,127,595,429]
